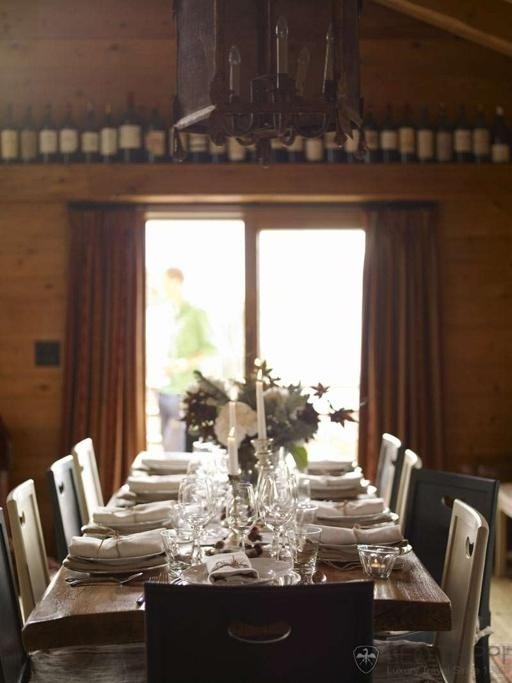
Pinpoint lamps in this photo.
[170,0,367,167]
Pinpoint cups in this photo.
[358,544,400,580]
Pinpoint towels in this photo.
[63,471,183,572]
[300,473,405,555]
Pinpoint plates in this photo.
[62,451,191,574]
[293,459,414,572]
[170,557,302,586]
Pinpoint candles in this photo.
[255,380,268,439]
[227,400,239,474]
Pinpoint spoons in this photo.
[65,572,144,586]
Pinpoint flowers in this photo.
[181,355,365,473]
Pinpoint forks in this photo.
[136,574,170,605]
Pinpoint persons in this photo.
[155,266,211,434]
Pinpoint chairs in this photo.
[0,430,146,681]
[143,578,377,682]
[355,428,502,683]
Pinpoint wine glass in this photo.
[159,439,322,574]
